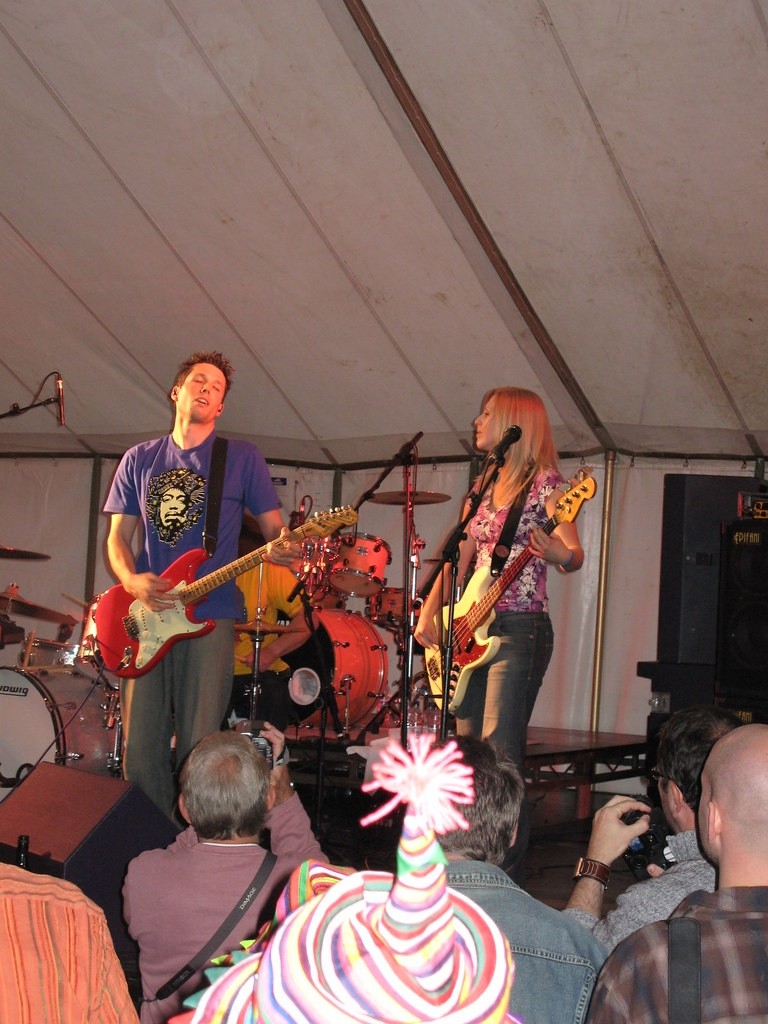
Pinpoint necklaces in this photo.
[489,466,535,521]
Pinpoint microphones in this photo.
[56,374,66,426]
[487,425,522,466]
[299,498,306,525]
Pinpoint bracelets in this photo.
[560,549,575,568]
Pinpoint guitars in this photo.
[424,476,597,712]
[95,504,359,680]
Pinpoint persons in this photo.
[103,352,303,831]
[414,387,584,890]
[565,695,768,1024]
[424,737,609,1024]
[121,722,329,1024]
[0,862,142,1024]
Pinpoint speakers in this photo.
[658,472,768,729]
[0,761,182,953]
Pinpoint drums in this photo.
[0,594,121,804]
[231,531,421,729]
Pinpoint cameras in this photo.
[241,732,274,771]
[622,794,674,881]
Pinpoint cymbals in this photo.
[233,619,308,637]
[366,491,451,506]
[0,591,79,624]
[423,557,442,563]
[0,546,51,560]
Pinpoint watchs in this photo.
[572,856,613,889]
